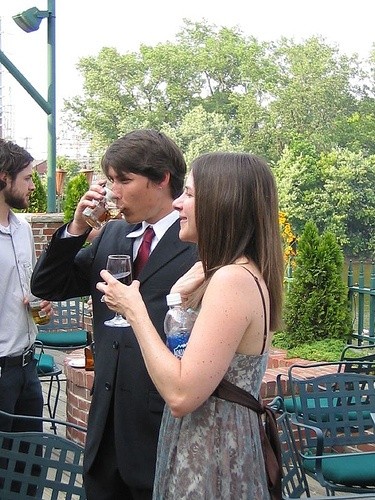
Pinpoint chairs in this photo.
[0,299,375,500]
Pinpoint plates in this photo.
[69,357,85,367]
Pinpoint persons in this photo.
[96,151,282,500]
[30,128,201,500]
[0,138,52,498]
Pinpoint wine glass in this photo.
[104,256,133,328]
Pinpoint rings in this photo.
[100,296,105,303]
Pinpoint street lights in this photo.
[0,0,58,214]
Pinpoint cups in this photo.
[82,186,126,231]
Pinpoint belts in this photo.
[1,350,35,369]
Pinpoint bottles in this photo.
[163,293,194,358]
[84,332,95,371]
[20,261,51,325]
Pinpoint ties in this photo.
[132,228,154,276]
